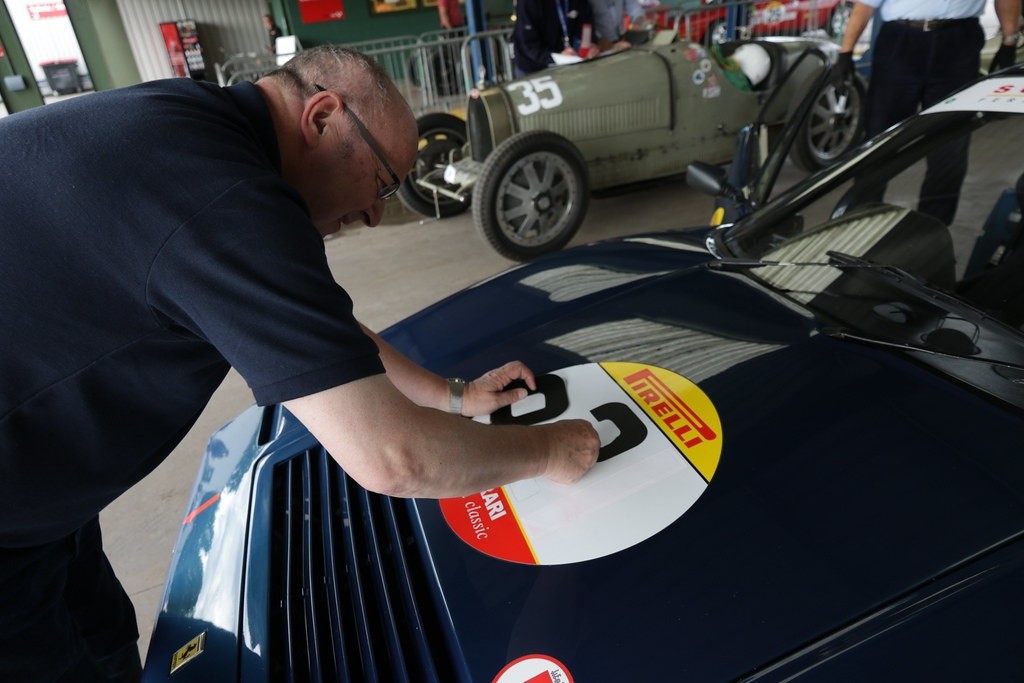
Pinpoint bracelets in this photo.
[1003,33,1024,47]
[448,377,467,414]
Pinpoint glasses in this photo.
[314,83,402,202]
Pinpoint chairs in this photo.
[961,171,1024,330]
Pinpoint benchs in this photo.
[714,39,786,91]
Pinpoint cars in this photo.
[621,0,856,49]
[395,38,868,265]
[135,60,1024,683]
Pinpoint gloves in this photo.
[988,40,1016,74]
[832,51,855,96]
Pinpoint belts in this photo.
[895,17,979,32]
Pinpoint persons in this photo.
[438,0,464,39]
[262,15,283,52]
[1,48,599,682]
[829,0,1024,228]
[507,0,647,80]
